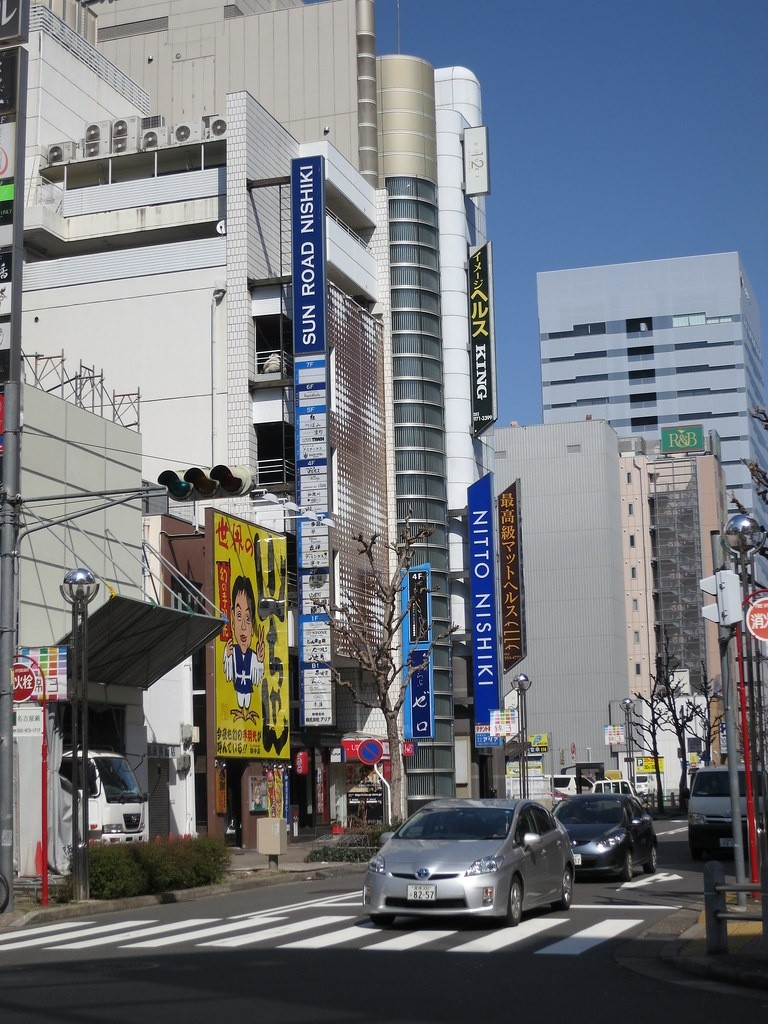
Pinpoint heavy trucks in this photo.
[59,735,161,848]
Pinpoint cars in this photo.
[544,775,594,795]
[553,792,657,881]
[362,798,575,927]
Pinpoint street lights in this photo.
[721,516,766,895]
[511,674,532,800]
[620,698,635,787]
[60,568,99,903]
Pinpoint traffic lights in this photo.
[699,570,743,627]
[158,465,255,501]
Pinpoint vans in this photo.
[590,780,642,803]
[683,766,768,858]
